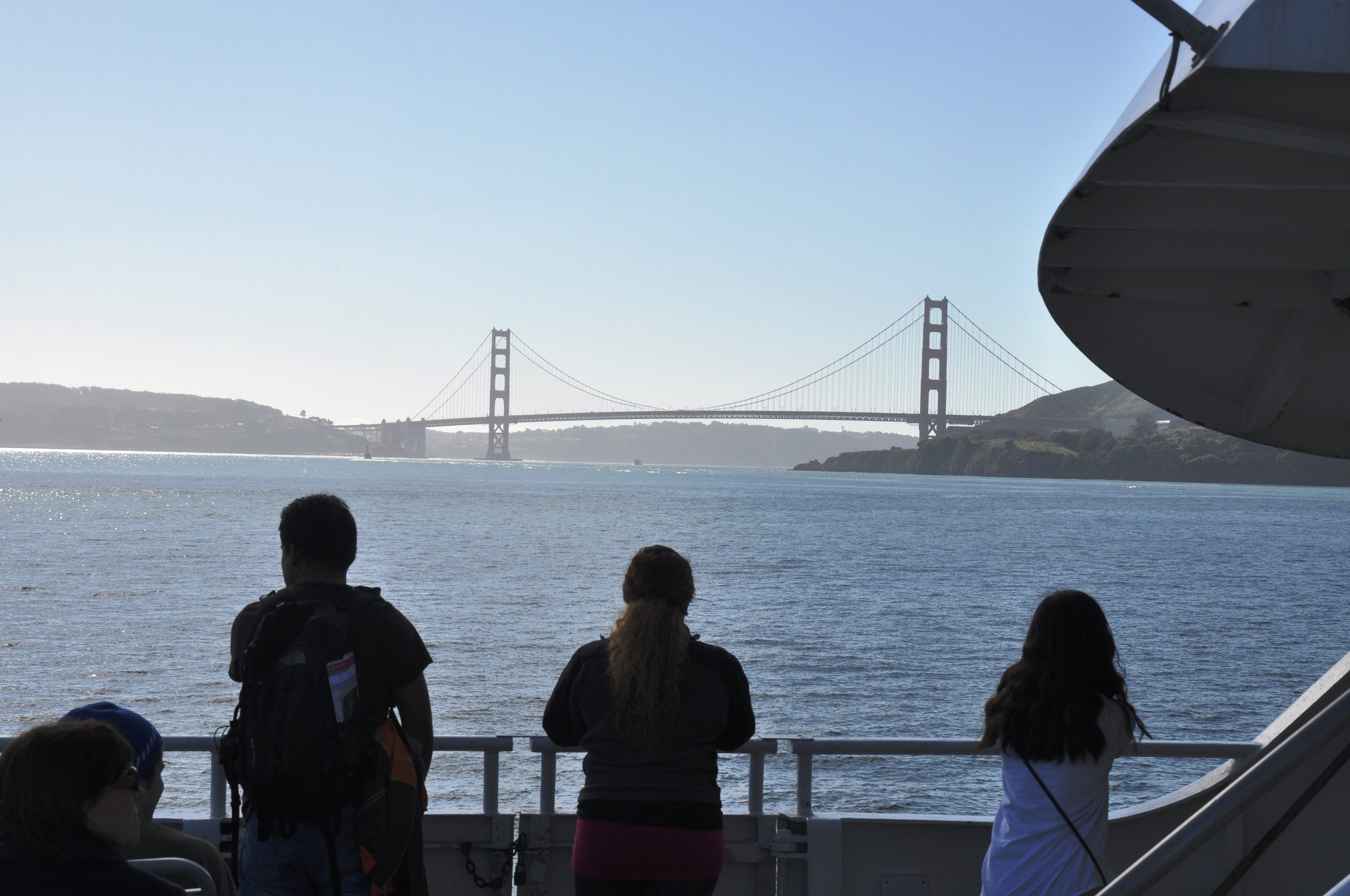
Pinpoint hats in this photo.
[55,701,163,780]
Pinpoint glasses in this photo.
[89,767,139,811]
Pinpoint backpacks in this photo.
[210,586,371,895]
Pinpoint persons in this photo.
[53,700,238,896]
[218,495,440,894]
[537,541,762,896]
[0,720,189,895]
[973,589,1158,895]
